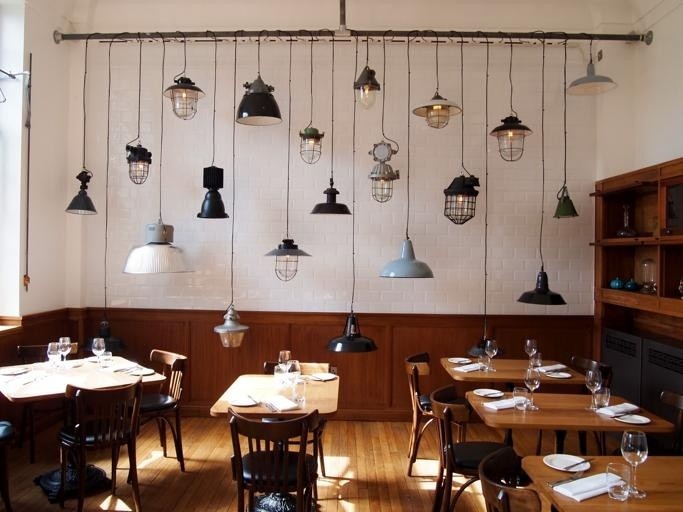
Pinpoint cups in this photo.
[477,352,542,372]
[605,461,629,501]
[512,387,528,411]
[97,351,114,374]
[594,387,610,408]
[274,360,308,406]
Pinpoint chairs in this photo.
[227,361,339,511]
[0,343,186,511]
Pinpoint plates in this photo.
[614,413,651,425]
[0,365,30,375]
[545,372,571,380]
[543,453,591,474]
[228,398,262,408]
[309,372,336,382]
[131,368,156,377]
[472,387,503,399]
[447,356,471,364]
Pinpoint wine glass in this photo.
[523,367,540,412]
[619,429,648,500]
[584,367,603,412]
[46,336,106,367]
[286,360,302,378]
[483,338,538,372]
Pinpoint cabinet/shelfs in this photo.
[591,157,683,387]
